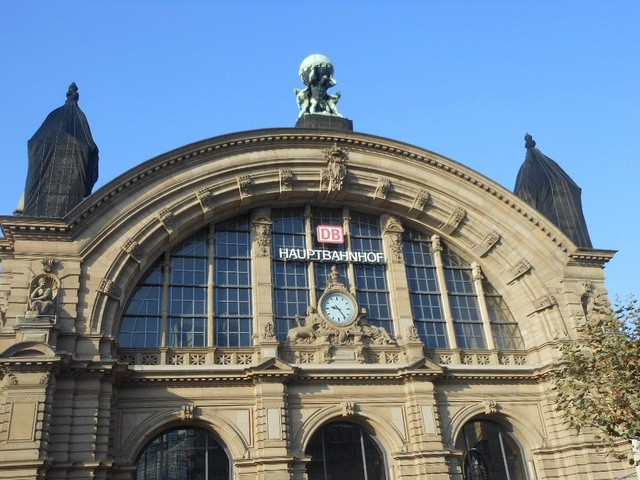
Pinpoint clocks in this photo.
[323,293,354,323]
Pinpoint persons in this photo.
[355,308,396,345]
[30,277,56,315]
[284,306,321,346]
